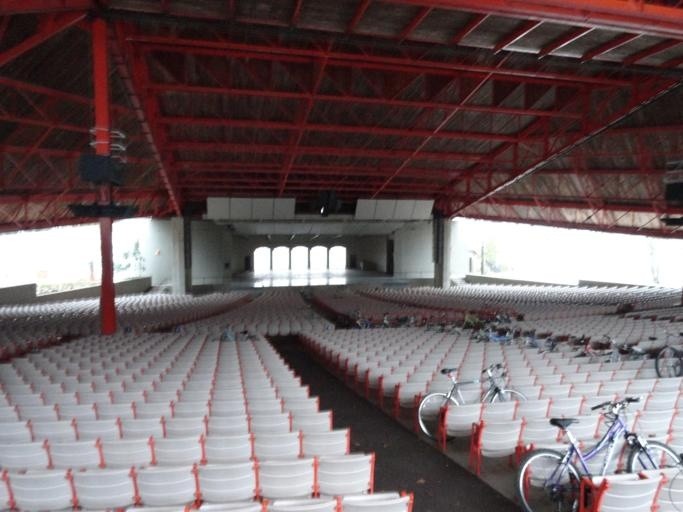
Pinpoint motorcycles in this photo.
[613,300,637,314]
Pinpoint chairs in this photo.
[0,271,682,352]
[302,325,681,510]
[1,330,416,510]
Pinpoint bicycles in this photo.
[415,362,527,440]
[516,393,683,511]
[217,322,260,343]
[511,326,683,377]
[350,308,516,344]
